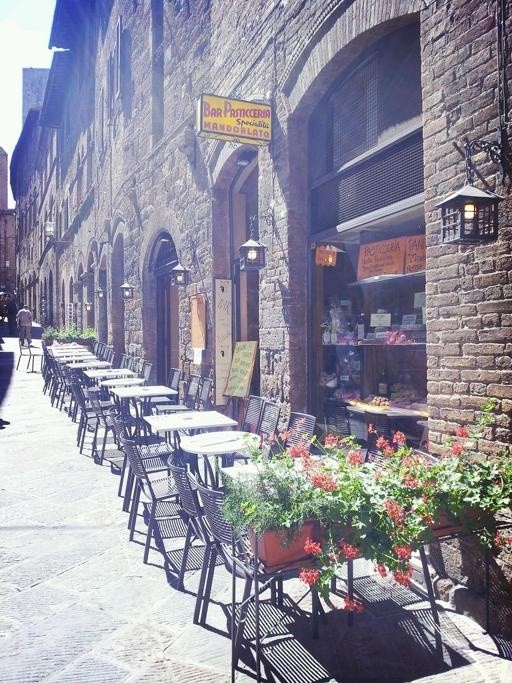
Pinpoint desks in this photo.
[143,411,238,436]
[57,355,98,363]
[112,386,179,450]
[181,430,262,468]
[66,361,112,369]
[100,378,146,387]
[83,368,137,378]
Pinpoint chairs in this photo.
[107,395,438,640]
[42,340,219,467]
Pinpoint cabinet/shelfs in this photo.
[391,312,401,331]
[378,372,388,398]
[357,312,365,341]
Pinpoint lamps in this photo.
[434,136,510,245]
[84,274,134,312]
[169,245,198,286]
[237,207,278,273]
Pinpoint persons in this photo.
[0,288,24,336]
[15,304,34,347]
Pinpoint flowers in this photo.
[203,391,511,614]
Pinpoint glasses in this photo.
[237,207,278,273]
[84,274,134,312]
[169,245,198,286]
[434,136,510,245]
